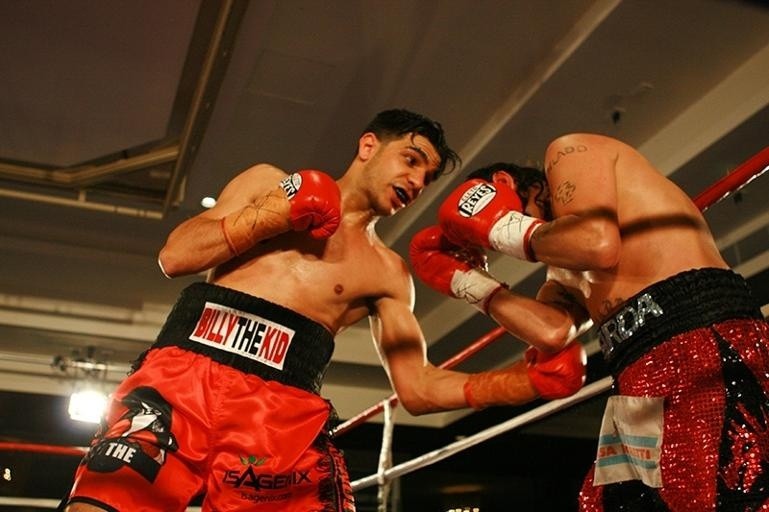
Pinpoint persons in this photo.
[63,107,590,510]
[410,129,769,512]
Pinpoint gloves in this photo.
[409,224,511,320]
[222,168,343,255]
[464,337,587,410]
[438,179,544,265]
